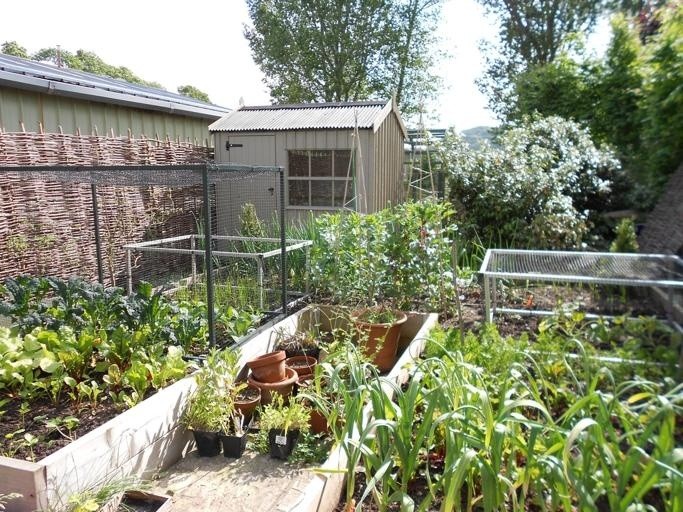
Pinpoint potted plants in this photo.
[181,210,409,461]
